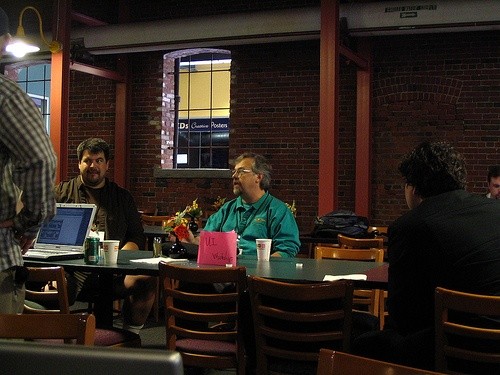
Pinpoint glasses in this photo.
[231,169,253,175]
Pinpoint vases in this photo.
[188,217,198,232]
[168,237,187,259]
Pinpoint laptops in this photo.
[22,203,98,261]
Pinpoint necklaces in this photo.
[235,193,270,234]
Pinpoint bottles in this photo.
[153,237,162,258]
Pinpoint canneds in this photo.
[84,237,99,265]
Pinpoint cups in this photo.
[255,238,272,261]
[103,240,120,266]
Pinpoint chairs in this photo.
[0,198,500,375]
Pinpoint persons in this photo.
[24,136,157,335]
[0,72,57,341]
[177,153,302,333]
[479,166,500,199]
[351,139,500,375]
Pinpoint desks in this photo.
[19,247,389,334]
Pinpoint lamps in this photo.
[5,7,59,58]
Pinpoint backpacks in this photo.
[311,209,370,239]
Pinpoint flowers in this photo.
[164,209,190,241]
[283,200,296,216]
[184,199,203,221]
